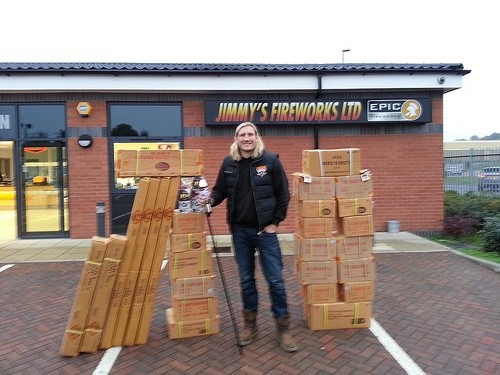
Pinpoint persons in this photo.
[209,122,298,352]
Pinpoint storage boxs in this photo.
[292,148,375,331]
[59,150,219,358]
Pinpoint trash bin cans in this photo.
[386,221,400,233]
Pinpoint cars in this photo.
[445,167,462,175]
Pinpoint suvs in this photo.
[478,166,500,190]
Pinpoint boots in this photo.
[235,309,257,346]
[275,316,298,351]
